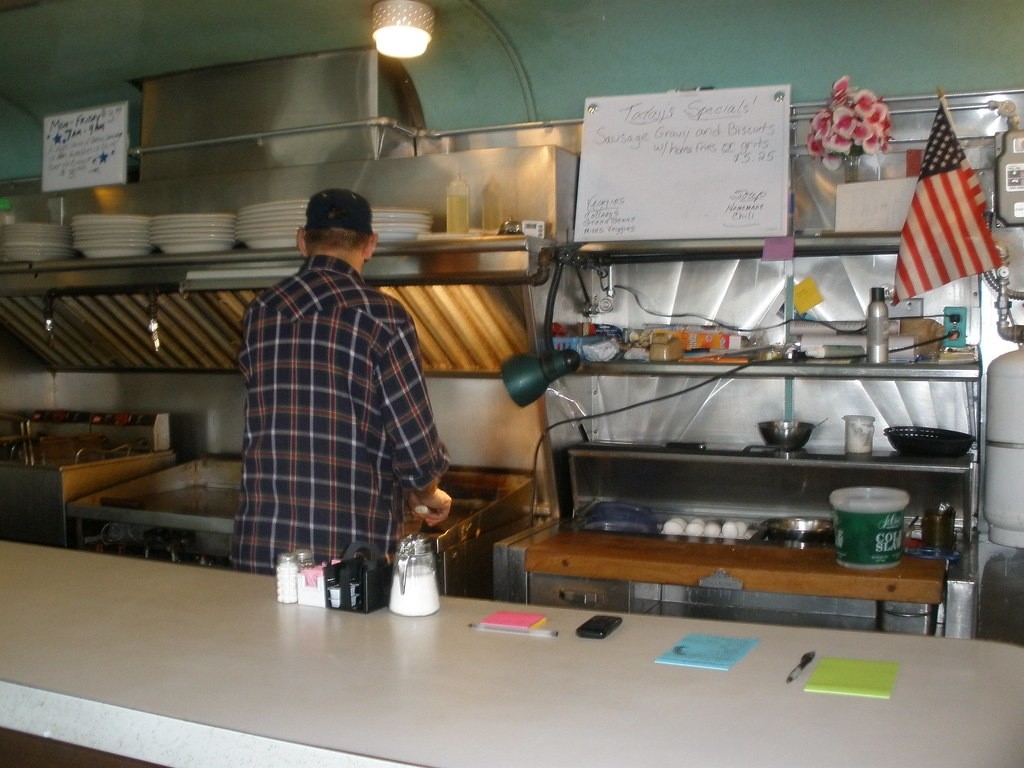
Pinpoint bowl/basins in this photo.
[756,419,814,451]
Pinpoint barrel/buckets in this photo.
[829,486,910,571]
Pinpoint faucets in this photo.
[0,412,31,449]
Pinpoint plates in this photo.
[0,201,434,263]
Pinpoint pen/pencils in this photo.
[786,649,816,684]
[467,620,559,637]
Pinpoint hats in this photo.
[306,188,371,230]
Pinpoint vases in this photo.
[843,154,859,183]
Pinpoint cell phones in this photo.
[575,615,623,639]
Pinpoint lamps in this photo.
[371,0,436,60]
[501,259,582,408]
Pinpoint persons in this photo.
[230,188,452,576]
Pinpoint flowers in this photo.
[804,75,894,172]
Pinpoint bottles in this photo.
[844,416,875,454]
[275,546,314,604]
[481,174,502,230]
[0,198,15,225]
[868,287,889,365]
[388,533,440,615]
[446,172,469,235]
[923,509,955,547]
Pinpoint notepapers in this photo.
[480,612,547,630]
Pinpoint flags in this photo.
[893,97,1002,305]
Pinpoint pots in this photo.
[578,498,664,534]
[761,516,834,540]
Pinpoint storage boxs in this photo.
[671,331,750,352]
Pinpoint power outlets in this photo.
[942,306,967,347]
